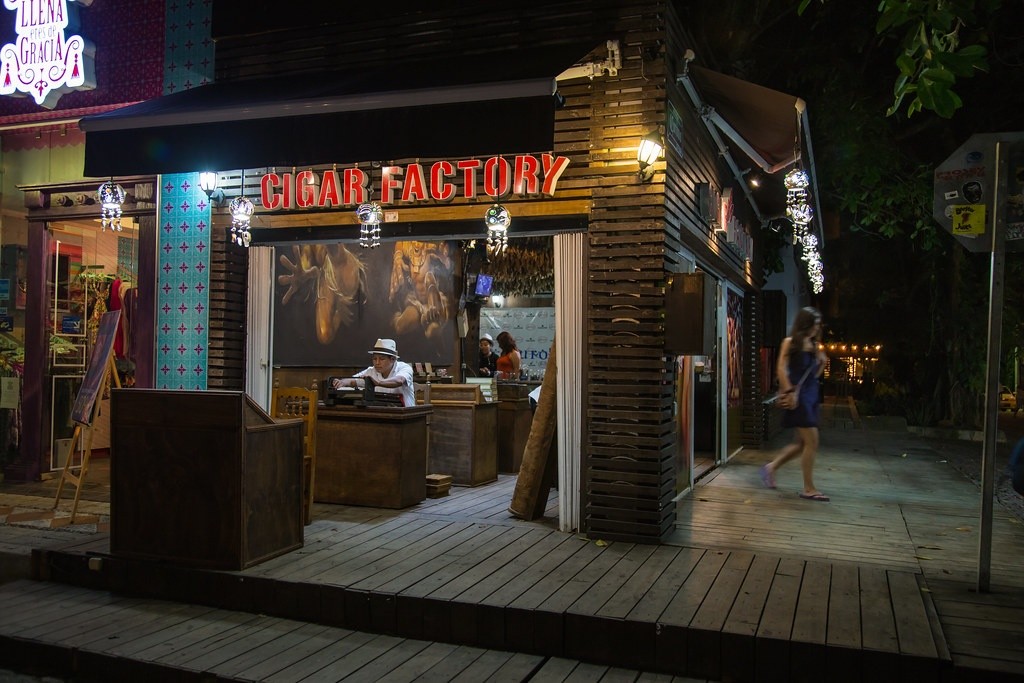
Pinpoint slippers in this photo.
[762,465,776,488]
[800,493,830,501]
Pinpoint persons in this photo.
[332,338,416,407]
[473,334,499,377]
[496,331,521,380]
[761,306,832,500]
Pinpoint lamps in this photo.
[801,222,821,261]
[229,169,255,248]
[812,274,824,294]
[790,193,814,245]
[97,177,127,233]
[198,169,225,208]
[783,136,809,219]
[356,162,383,250]
[485,155,512,260]
[807,261,823,281]
[636,123,665,183]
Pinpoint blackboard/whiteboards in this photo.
[70,308,121,425]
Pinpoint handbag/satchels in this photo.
[774,385,798,409]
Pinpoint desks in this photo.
[285,399,433,509]
[417,401,503,488]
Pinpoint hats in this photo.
[480,333,494,347]
[368,338,400,358]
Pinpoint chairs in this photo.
[413,381,432,476]
[270,377,319,526]
